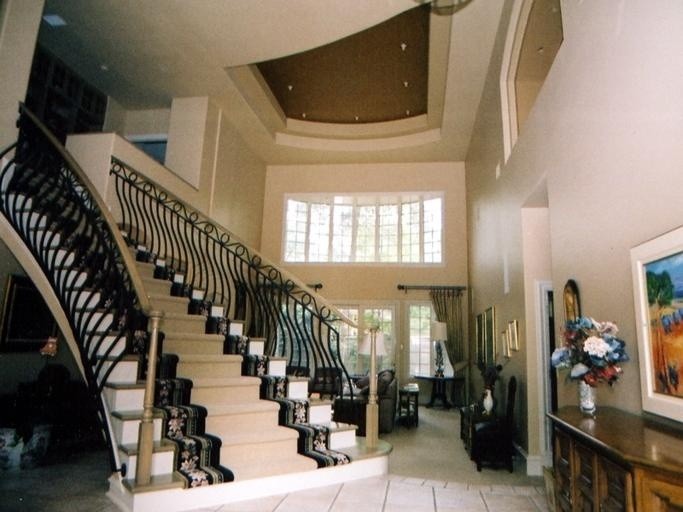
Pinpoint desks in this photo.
[399,381,419,424]
[413,374,463,409]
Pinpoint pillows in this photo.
[357,368,398,388]
[360,372,393,396]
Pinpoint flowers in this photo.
[551,316,628,385]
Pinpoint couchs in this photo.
[336,364,398,435]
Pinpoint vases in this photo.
[482,390,494,416]
[577,378,597,414]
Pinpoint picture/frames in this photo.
[501,329,512,358]
[474,312,484,366]
[484,305,495,371]
[629,223,682,422]
[507,319,518,351]
[563,279,580,323]
[0,274,58,355]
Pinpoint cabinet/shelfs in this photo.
[536,403,682,510]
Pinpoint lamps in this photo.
[430,321,448,377]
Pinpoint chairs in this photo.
[459,372,495,441]
[307,367,342,400]
[471,374,518,471]
